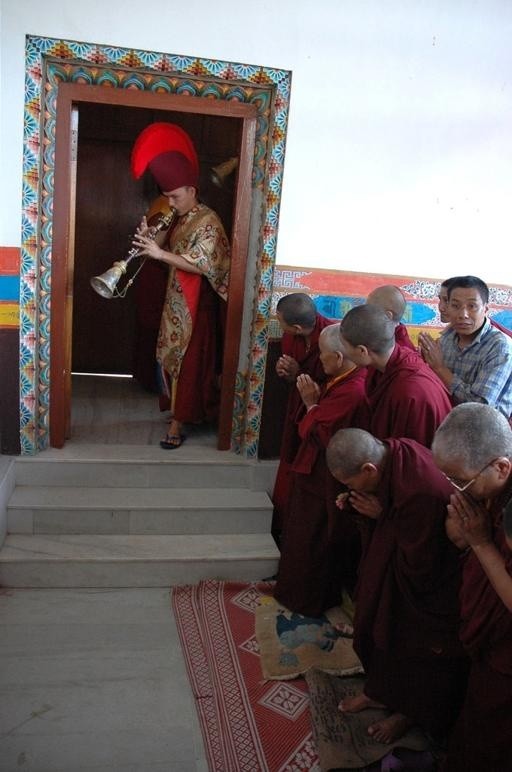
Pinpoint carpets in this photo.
[172,576,422,772]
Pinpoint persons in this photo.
[130,186,231,447]
[133,193,178,396]
[261,277,511,770]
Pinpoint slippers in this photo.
[159,432,186,450]
